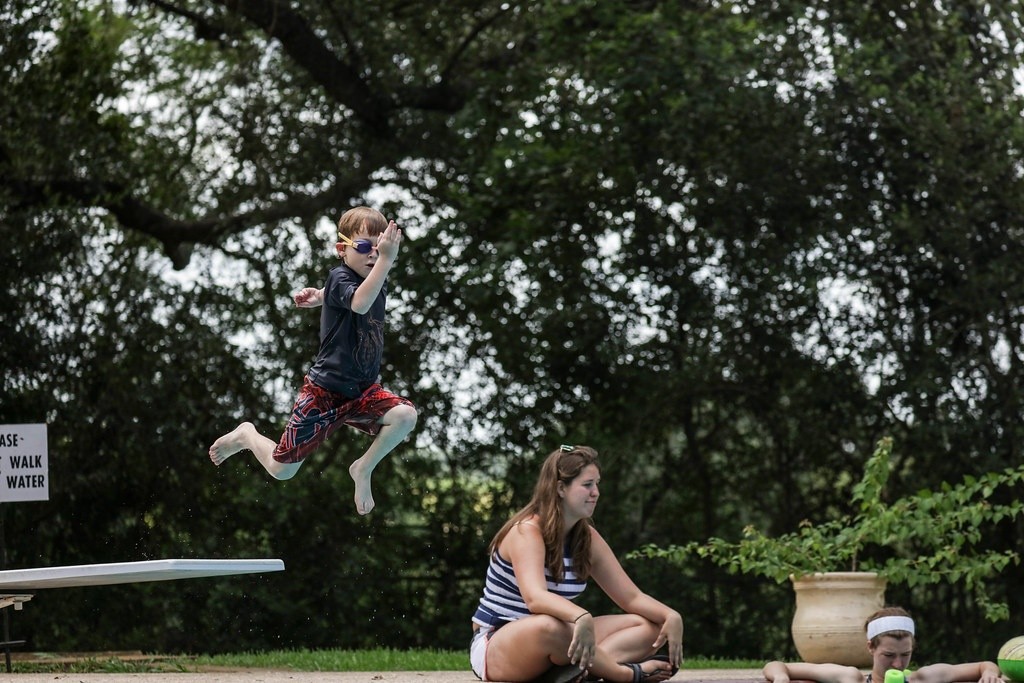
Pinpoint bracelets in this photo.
[573,611,588,624]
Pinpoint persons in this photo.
[209,207,417,515]
[762,608,1005,683]
[469,445,684,683]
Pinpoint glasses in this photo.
[338,232,379,256]
[559,445,574,460]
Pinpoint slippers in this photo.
[625,655,679,683]
[533,664,585,683]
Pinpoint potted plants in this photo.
[626,436,1024,667]
